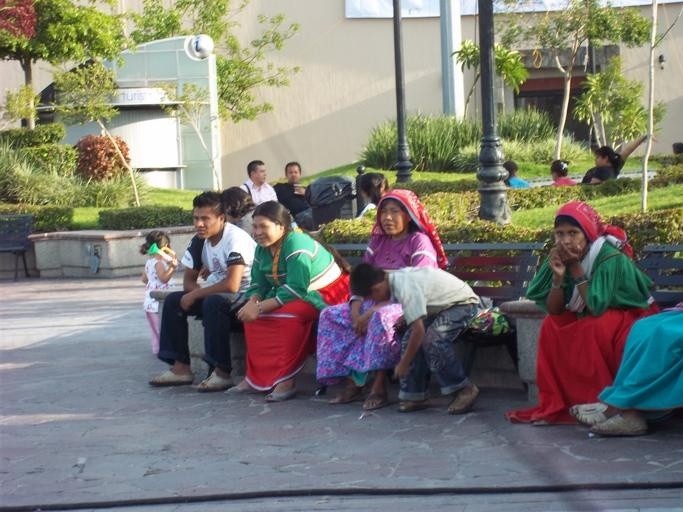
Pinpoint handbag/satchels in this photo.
[470,307,510,336]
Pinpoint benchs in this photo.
[150,240,544,405]
[0,213,33,281]
[632,239,682,312]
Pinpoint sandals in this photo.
[148,370,194,385]
[570,402,608,424]
[265,376,297,401]
[398,400,431,412]
[363,392,389,409]
[196,370,232,391]
[224,380,267,395]
[590,412,648,435]
[329,387,367,404]
[447,384,481,414]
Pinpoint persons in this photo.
[501,158,529,189]
[504,198,659,429]
[565,298,683,438]
[539,158,579,186]
[575,131,659,184]
[140,160,485,415]
